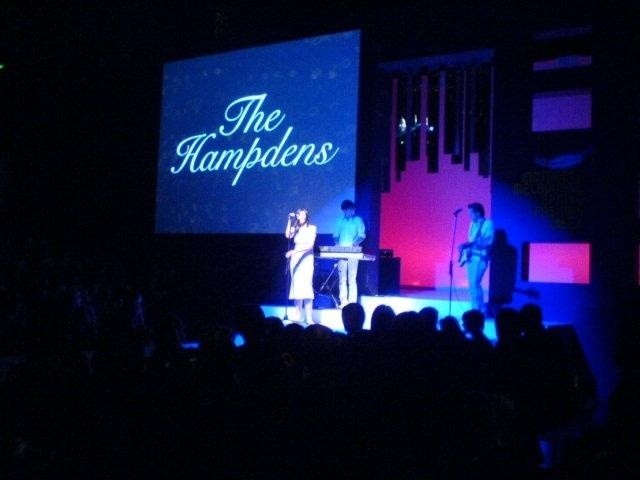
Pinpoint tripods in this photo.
[282,221,309,325]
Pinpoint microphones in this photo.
[452,209,462,215]
[288,213,296,218]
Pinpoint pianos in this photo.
[315,246,376,262]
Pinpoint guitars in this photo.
[457,244,487,267]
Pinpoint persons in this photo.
[285,207,317,324]
[333,198,366,310]
[0,295,604,478]
[459,202,495,311]
